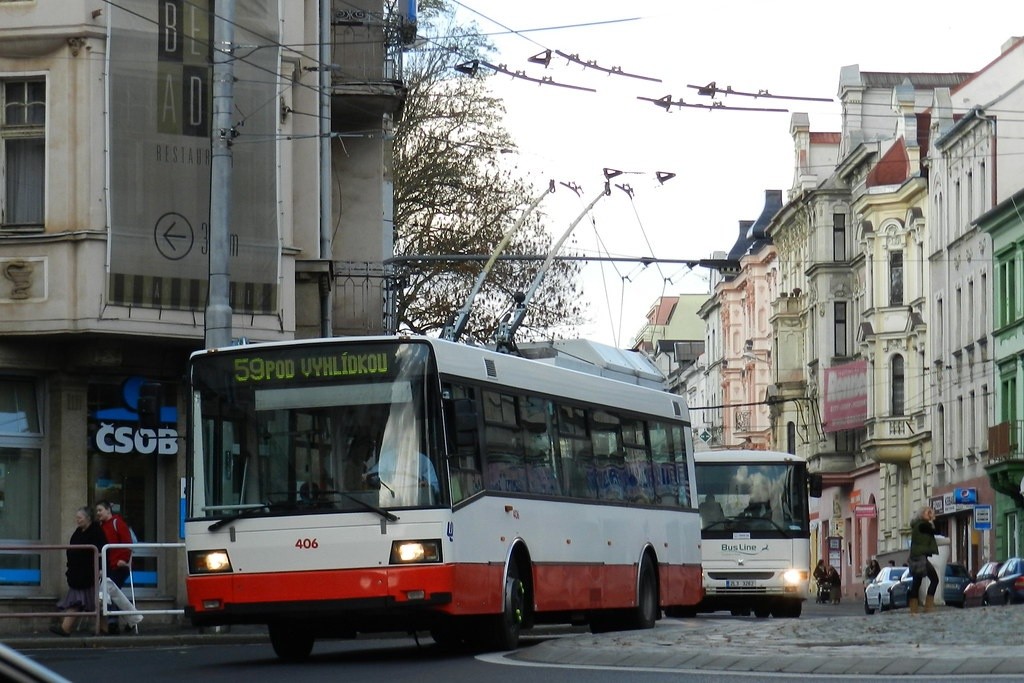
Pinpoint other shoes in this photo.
[50,626,70,637]
[93,628,106,636]
[107,622,121,636]
[124,622,136,633]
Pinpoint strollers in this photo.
[817,578,831,603]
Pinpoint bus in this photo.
[671,449,824,620]
[185,184,704,660]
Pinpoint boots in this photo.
[910,598,920,614]
[924,594,938,612]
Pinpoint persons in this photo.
[698,493,726,531]
[813,559,842,605]
[908,505,939,618]
[342,433,440,505]
[48,499,143,638]
[746,484,792,520]
[865,559,908,588]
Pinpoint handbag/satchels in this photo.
[113,518,137,553]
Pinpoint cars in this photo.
[962,562,1002,606]
[888,562,970,610]
[981,557,1024,606]
[863,566,916,614]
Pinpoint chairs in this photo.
[748,496,770,506]
[699,501,724,529]
